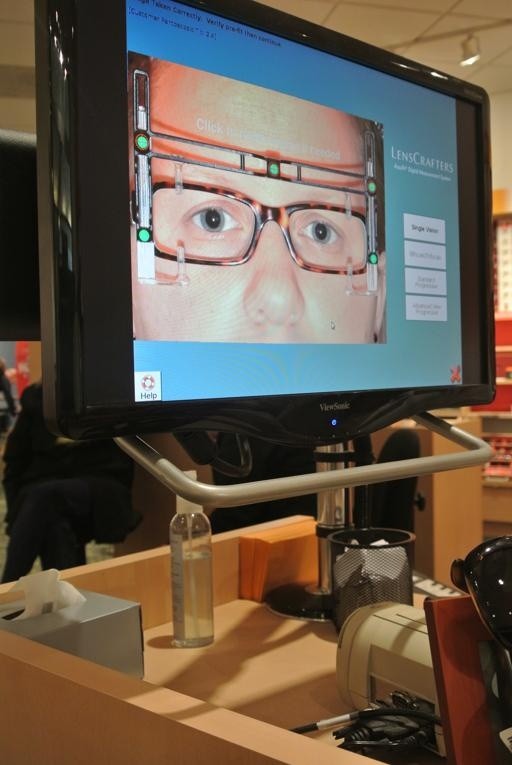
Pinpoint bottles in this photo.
[168,468,216,647]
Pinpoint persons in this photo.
[0,357,20,435]
[0,376,145,585]
[207,432,374,535]
[130,49,386,341]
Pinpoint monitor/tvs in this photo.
[34,0,496,451]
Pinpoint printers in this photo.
[332,597,456,762]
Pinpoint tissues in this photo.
[1,568,144,682]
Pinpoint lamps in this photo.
[457,28,482,68]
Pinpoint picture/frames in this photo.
[421,592,512,765]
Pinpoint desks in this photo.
[0,514,511,764]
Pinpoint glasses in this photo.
[128,181,380,276]
